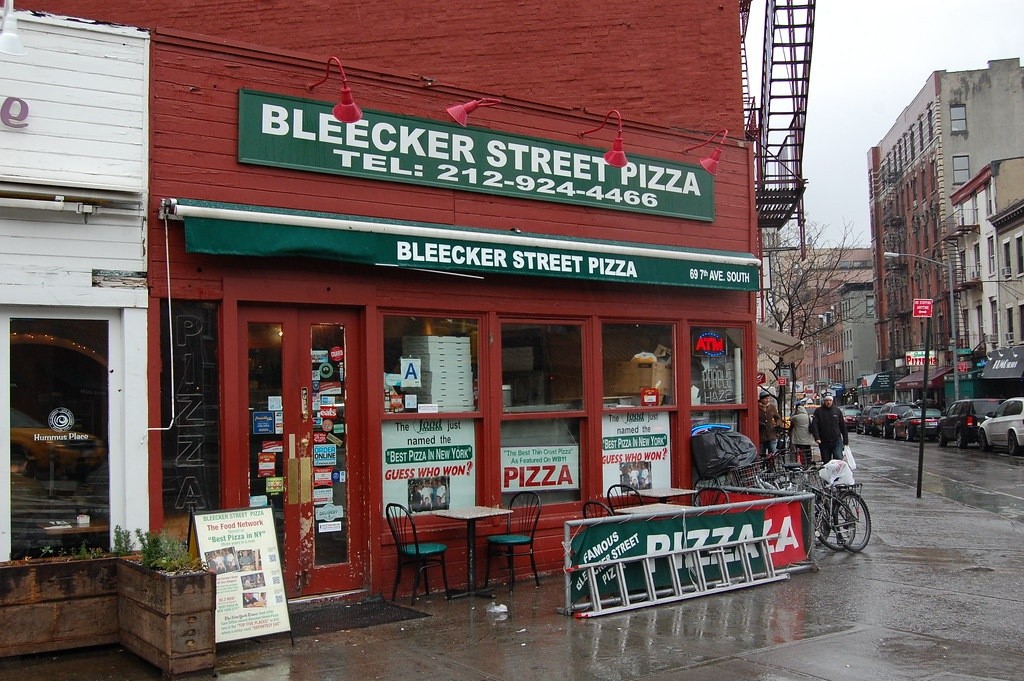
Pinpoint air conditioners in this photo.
[971,271,980,279]
[1001,267,1012,277]
[1005,333,1013,343]
[986,334,998,344]
[829,347,832,352]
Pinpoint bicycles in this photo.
[691,449,872,552]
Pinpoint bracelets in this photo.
[251,602,257,607]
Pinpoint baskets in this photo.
[728,460,766,494]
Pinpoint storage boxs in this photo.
[402,336,475,413]
[613,360,672,397]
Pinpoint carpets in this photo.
[260,599,433,641]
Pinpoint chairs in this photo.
[485,491,541,597]
[583,501,614,519]
[692,475,720,507]
[691,486,729,507]
[606,484,644,513]
[385,503,452,606]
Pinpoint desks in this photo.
[614,502,698,515]
[36,521,109,554]
[625,488,699,504]
[431,506,512,600]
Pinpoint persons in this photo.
[790,402,813,467]
[204,548,265,608]
[811,392,848,467]
[757,391,783,471]
[408,477,449,512]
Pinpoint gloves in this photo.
[774,424,780,429]
[762,423,767,429]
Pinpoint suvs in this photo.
[871,402,921,439]
[856,404,883,435]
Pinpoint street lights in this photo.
[816,344,822,406]
[884,252,959,403]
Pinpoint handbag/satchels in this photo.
[842,445,856,471]
[818,459,855,488]
[776,433,786,450]
[809,416,813,433]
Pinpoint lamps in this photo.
[683,128,728,175]
[445,97,502,129]
[305,57,364,124]
[577,110,628,168]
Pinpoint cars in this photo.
[977,397,1024,455]
[837,406,843,412]
[804,405,821,424]
[841,408,862,431]
[892,407,942,441]
[842,405,857,412]
[10,406,107,487]
[771,409,791,429]
[935,399,1007,449]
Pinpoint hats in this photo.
[823,391,833,400]
[759,391,770,399]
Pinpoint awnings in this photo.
[980,346,1024,378]
[756,323,804,366]
[177,195,759,291]
[895,367,954,388]
